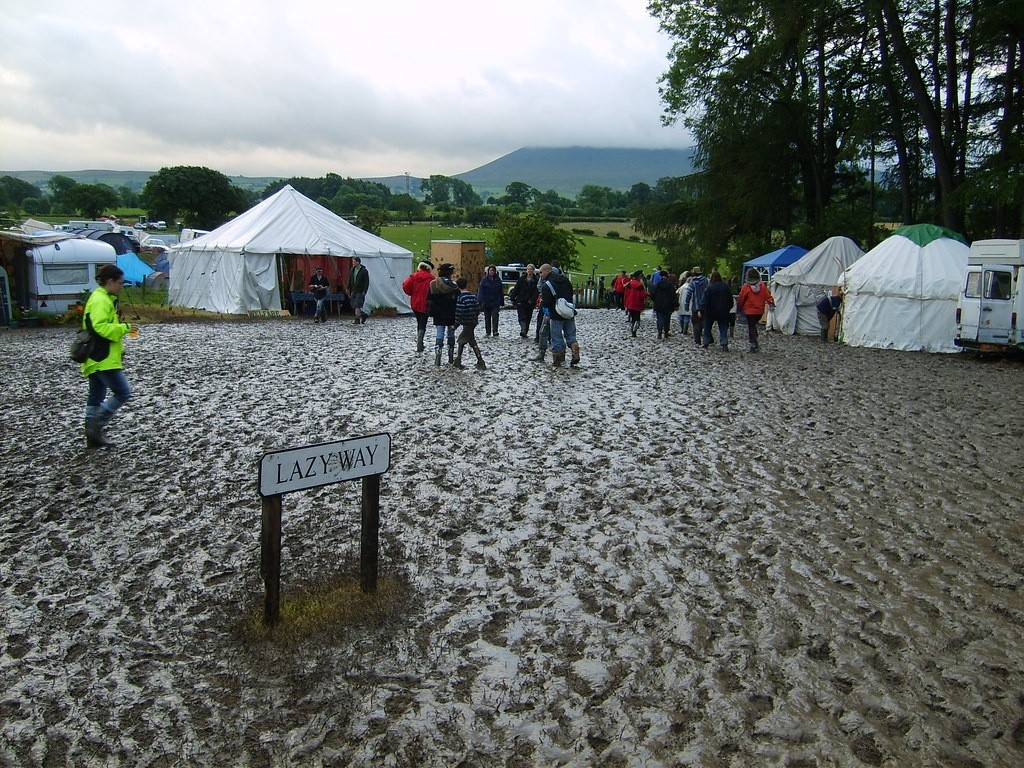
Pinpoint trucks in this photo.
[954,238,1024,360]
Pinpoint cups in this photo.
[130,324,140,340]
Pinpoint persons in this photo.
[347,257,369,324]
[426,263,461,365]
[600,266,736,352]
[737,269,775,352]
[449,278,486,369]
[816,293,842,343]
[309,267,329,323]
[540,264,580,366]
[511,261,566,362]
[402,261,437,352]
[83,265,140,448]
[478,266,504,335]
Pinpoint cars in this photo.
[118,220,211,252]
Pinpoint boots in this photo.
[528,354,544,361]
[448,347,454,363]
[361,311,369,323]
[561,355,566,361]
[486,324,491,337]
[474,346,483,362]
[616,304,837,353]
[85,403,118,448]
[519,321,528,338]
[493,322,500,336]
[455,347,462,363]
[353,318,360,324]
[416,330,425,351]
[552,352,560,366]
[570,343,580,364]
[435,348,441,366]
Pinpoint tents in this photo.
[169,184,414,314]
[838,224,971,353]
[767,237,866,335]
[21,218,53,235]
[64,228,155,286]
[742,245,809,288]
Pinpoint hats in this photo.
[691,267,702,273]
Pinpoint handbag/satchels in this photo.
[555,298,575,319]
[69,331,94,364]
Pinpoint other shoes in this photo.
[314,318,319,323]
[322,315,328,323]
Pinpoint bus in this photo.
[485,263,541,298]
